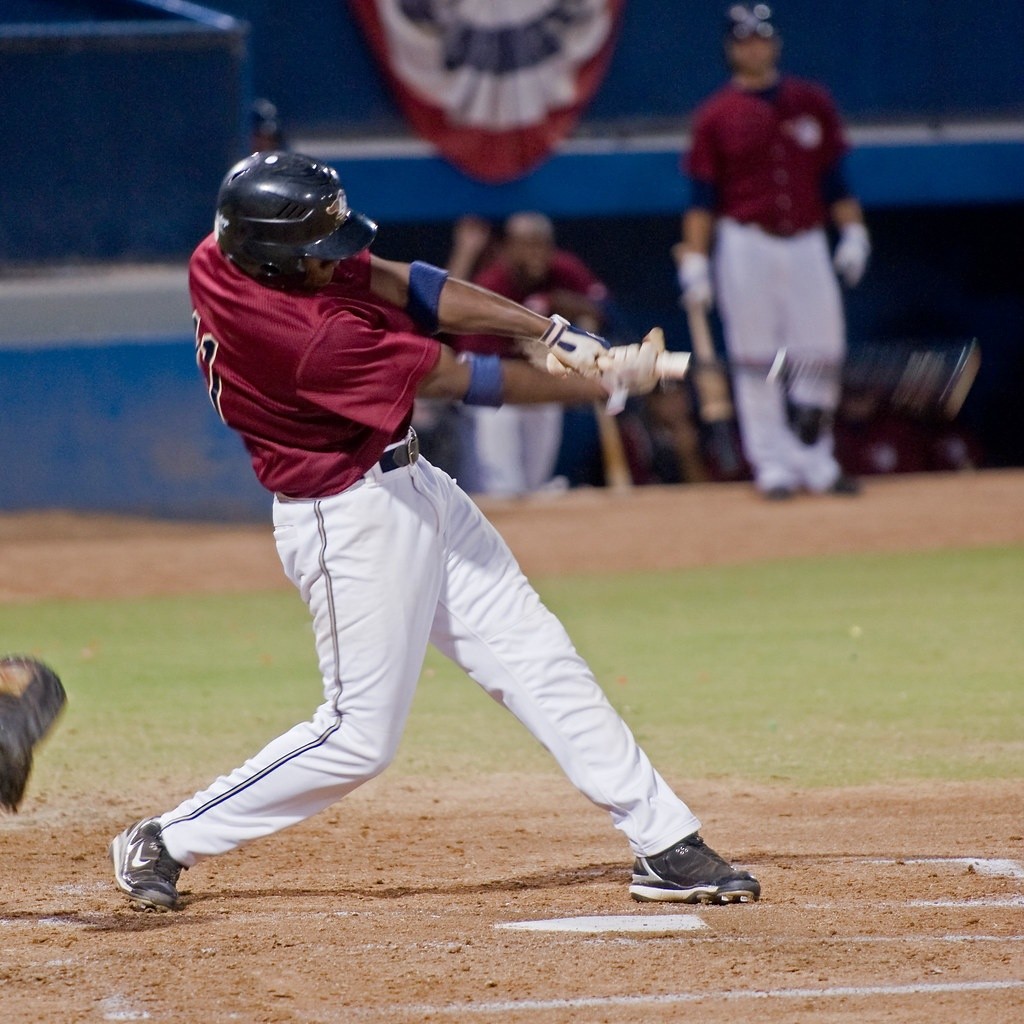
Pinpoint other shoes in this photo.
[830,470,858,495]
[761,484,788,500]
[0,654,67,820]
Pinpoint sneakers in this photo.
[107,816,182,912]
[629,831,761,906]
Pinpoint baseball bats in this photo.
[657,333,1006,425]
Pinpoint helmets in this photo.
[723,1,783,42]
[214,150,379,290]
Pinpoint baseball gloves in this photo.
[0,651,72,815]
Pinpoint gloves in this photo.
[834,223,870,287]
[537,314,610,379]
[603,328,665,416]
[677,252,714,309]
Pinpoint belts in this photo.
[380,426,420,474]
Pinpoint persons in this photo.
[448,214,605,493]
[110,151,760,910]
[682,19,871,499]
